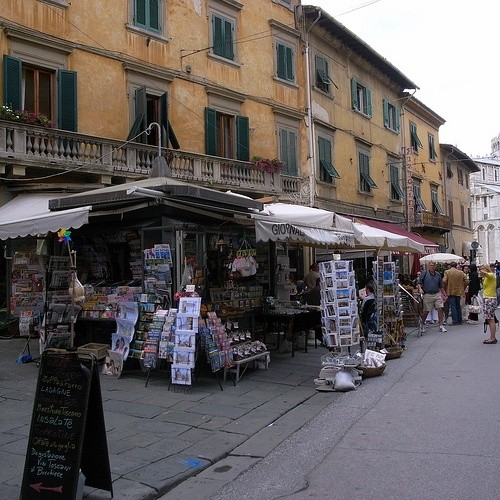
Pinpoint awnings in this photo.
[0,181,437,253]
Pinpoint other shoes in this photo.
[483,338,497,344]
[421,326,425,333]
[444,321,447,325]
[439,325,447,332]
[466,319,479,325]
[320,343,326,347]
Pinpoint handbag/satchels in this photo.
[69,276,84,297]
[467,297,481,314]
[484,316,499,333]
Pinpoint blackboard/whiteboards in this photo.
[18,351,113,500]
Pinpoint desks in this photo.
[263,310,321,357]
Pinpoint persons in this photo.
[203,258,224,296]
[104,337,129,374]
[305,262,500,345]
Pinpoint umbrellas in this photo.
[419,252,463,274]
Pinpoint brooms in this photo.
[18,334,32,363]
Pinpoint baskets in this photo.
[357,364,386,377]
[77,343,110,360]
[385,348,403,360]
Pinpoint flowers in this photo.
[252,155,280,174]
[0,106,52,128]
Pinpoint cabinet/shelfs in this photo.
[371,261,402,347]
[224,340,271,386]
[317,257,365,355]
